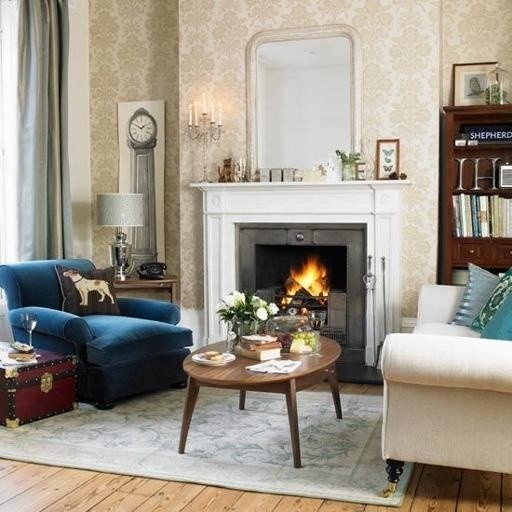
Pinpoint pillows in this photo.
[450,255,500,329]
[472,266,511,332]
[475,291,510,341]
[52,261,119,317]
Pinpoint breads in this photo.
[205,351,224,360]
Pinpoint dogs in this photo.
[62,268,115,306]
[218,158,234,182]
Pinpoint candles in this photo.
[188,104,194,130]
[200,92,210,118]
[216,100,223,129]
[209,103,216,126]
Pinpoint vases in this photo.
[223,320,260,353]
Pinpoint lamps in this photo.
[93,190,149,279]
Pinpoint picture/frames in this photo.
[374,137,401,179]
[446,62,501,109]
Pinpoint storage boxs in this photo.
[1,339,86,427]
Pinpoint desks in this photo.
[174,330,346,470]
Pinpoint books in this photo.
[449,193,512,238]
[233,333,283,361]
[1,341,37,365]
[246,358,304,375]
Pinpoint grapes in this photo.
[276,333,293,347]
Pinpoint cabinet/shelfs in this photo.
[439,100,511,288]
[106,271,184,311]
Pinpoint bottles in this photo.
[486,68,512,105]
[343,164,353,181]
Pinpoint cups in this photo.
[229,155,247,182]
[314,160,329,180]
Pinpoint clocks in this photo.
[126,107,160,272]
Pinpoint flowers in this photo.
[213,291,278,320]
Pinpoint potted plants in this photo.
[333,147,360,179]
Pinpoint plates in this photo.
[191,351,238,367]
[241,334,279,346]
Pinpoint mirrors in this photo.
[242,25,365,178]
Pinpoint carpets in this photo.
[0,373,420,509]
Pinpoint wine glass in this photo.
[307,309,326,358]
[19,307,40,352]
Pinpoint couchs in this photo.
[0,253,196,412]
[369,280,512,502]
